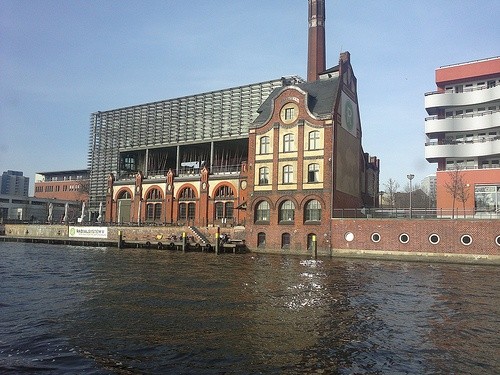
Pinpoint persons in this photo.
[162,228,232,245]
